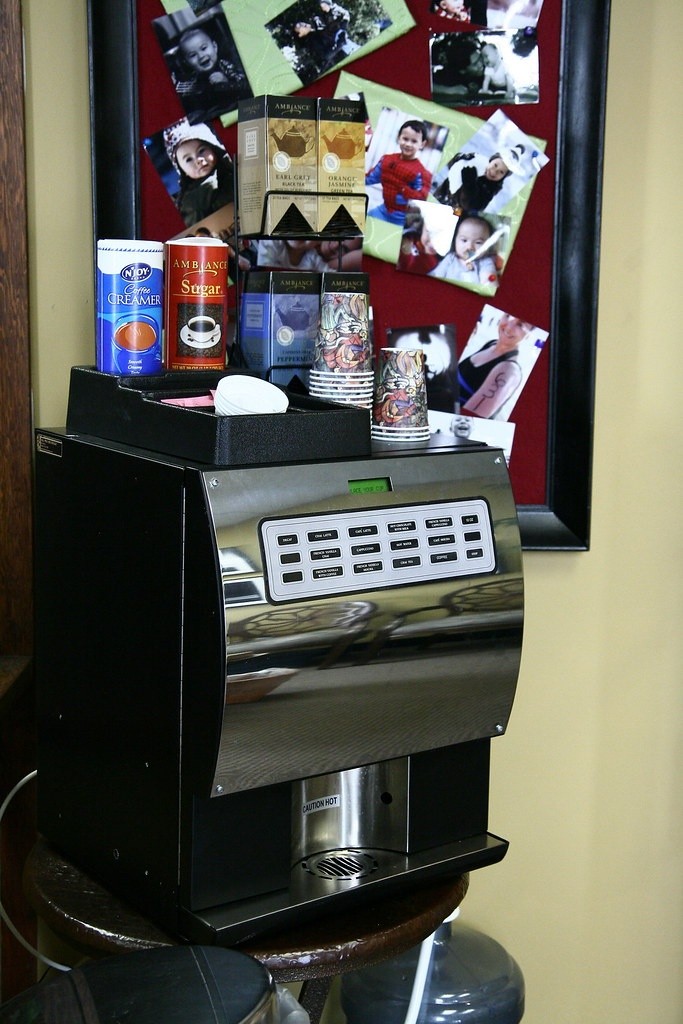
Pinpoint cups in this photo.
[309,292,430,442]
[111,313,161,376]
[187,316,220,342]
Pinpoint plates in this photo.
[180,325,221,348]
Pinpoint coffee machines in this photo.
[35,427,524,950]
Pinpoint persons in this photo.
[292,0,351,76]
[171,22,252,124]
[439,0,464,14]
[458,312,536,421]
[163,116,236,225]
[183,227,363,272]
[481,43,514,100]
[364,121,513,284]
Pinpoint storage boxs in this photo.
[317,271,370,297]
[314,96,369,237]
[241,270,320,397]
[234,93,319,235]
[68,362,378,466]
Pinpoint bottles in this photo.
[341,905,524,1024]
